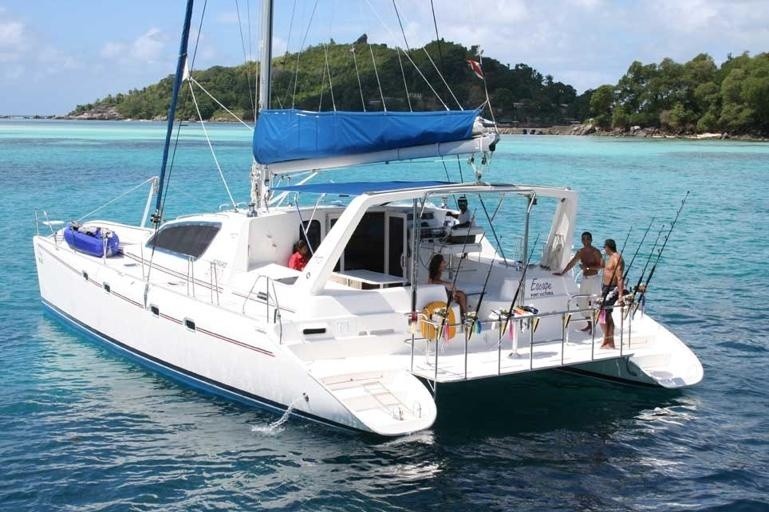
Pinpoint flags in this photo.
[467,58,486,81]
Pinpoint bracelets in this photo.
[619,294,622,298]
[586,266,590,271]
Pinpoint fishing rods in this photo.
[500,233,541,336]
[589,191,690,334]
[467,235,503,340]
[479,193,505,245]
[438,209,477,337]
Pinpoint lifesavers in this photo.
[421,302,456,341]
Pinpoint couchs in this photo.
[467,276,572,322]
[329,285,462,335]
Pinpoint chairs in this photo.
[432,226,485,269]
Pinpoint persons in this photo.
[444,196,475,245]
[289,239,309,273]
[599,239,627,350]
[552,231,606,334]
[428,254,468,325]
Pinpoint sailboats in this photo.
[32,0,705,439]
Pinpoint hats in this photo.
[458,197,467,201]
